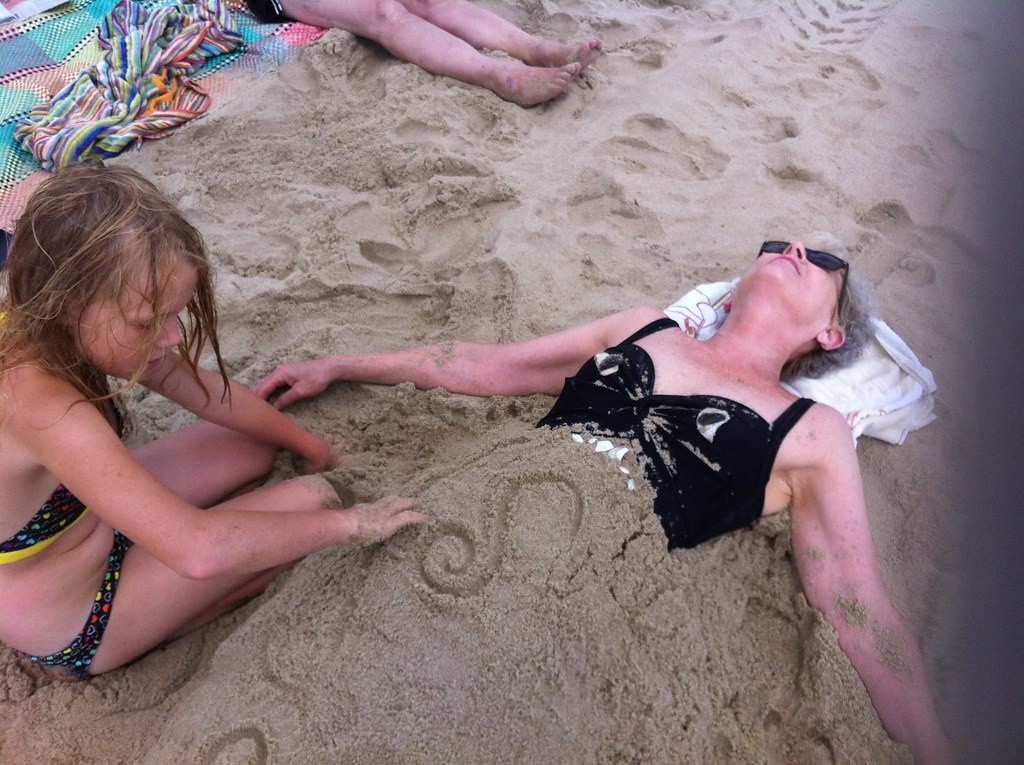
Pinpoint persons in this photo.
[250,240,951,765]
[0,160,430,680]
[243,1,604,108]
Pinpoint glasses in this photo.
[756,241,849,318]
